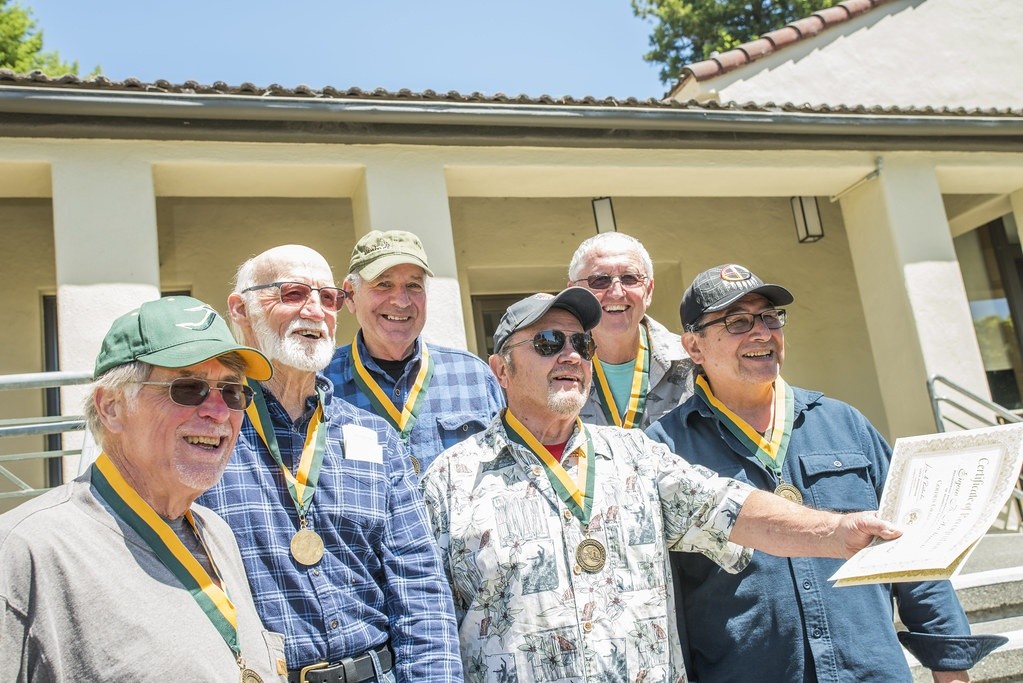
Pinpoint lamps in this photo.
[790,195,825,243]
[590,196,618,235]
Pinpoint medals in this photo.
[409,455,420,475]
[290,529,324,565]
[239,669,265,683]
[774,484,803,505]
[576,539,607,572]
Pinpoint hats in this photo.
[493,286,602,354]
[679,263,794,332]
[349,230,434,282]
[93,297,274,381]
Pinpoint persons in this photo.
[0,296,288,683]
[567,231,698,432]
[643,264,1009,683]
[193,245,465,683]
[417,286,903,683]
[316,230,507,485]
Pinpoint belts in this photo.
[288,644,393,683]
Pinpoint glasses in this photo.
[242,282,349,313]
[502,329,598,361]
[573,273,649,289]
[689,309,786,334]
[117,377,257,411]
